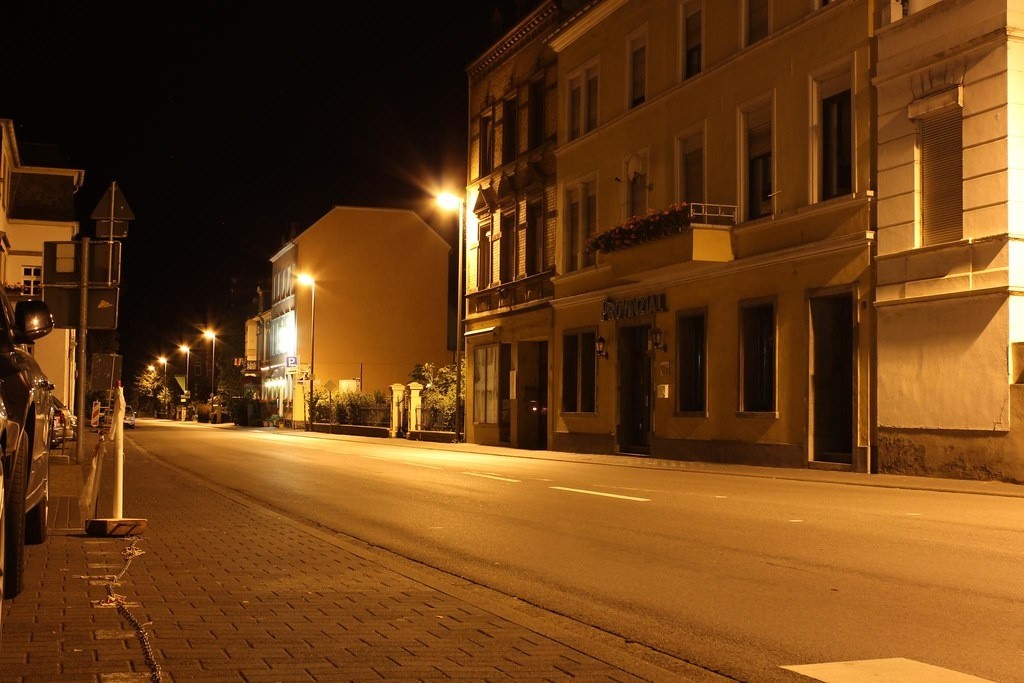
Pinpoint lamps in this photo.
[651,325,667,354]
[594,335,608,360]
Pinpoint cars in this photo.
[123,407,137,429]
[95,407,108,417]
[104,409,113,422]
[0,279,55,599]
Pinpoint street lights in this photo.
[150,366,155,371]
[182,347,190,390]
[299,274,315,432]
[159,358,167,397]
[438,193,463,442]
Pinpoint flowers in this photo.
[265,413,285,424]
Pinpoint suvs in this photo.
[50,392,73,448]
[68,410,77,442]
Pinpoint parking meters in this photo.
[205,331,215,402]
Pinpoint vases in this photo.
[263,420,284,428]
